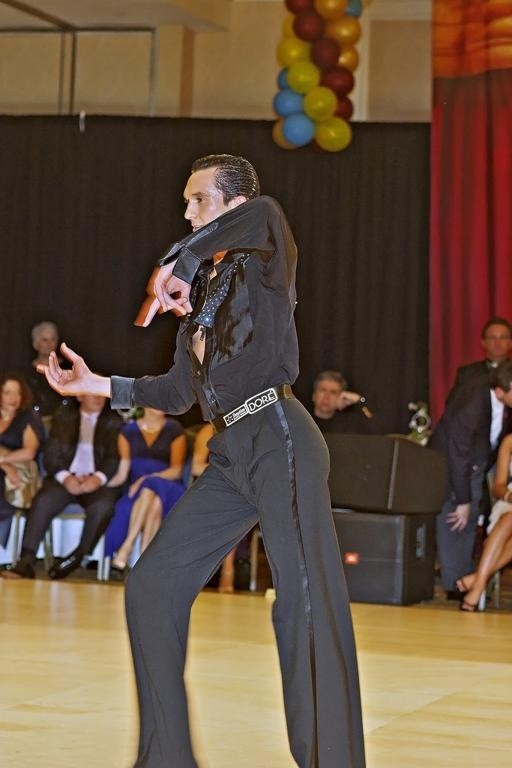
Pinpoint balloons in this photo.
[293,13,323,43]
[321,69,355,97]
[315,119,351,151]
[313,0,344,19]
[344,0,363,19]
[277,67,292,91]
[276,38,311,65]
[311,39,340,70]
[273,92,304,118]
[332,96,352,122]
[286,65,320,95]
[335,44,359,70]
[304,86,338,122]
[273,119,296,150]
[324,13,362,46]
[281,16,294,39]
[284,0,313,16]
[282,113,317,146]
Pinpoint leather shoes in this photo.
[447,590,464,600]
[48,554,81,580]
[1,559,35,578]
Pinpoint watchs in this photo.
[355,395,368,411]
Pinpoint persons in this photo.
[309,372,372,434]
[425,360,511,601]
[0,374,43,549]
[23,322,74,418]
[1,394,123,580]
[102,408,188,579]
[456,434,511,613]
[446,317,512,406]
[193,421,234,592]
[35,153,366,768]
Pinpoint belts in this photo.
[212,385,295,433]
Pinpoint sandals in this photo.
[455,578,468,594]
[461,597,480,613]
[111,561,128,579]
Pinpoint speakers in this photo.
[330,510,435,606]
[325,433,445,516]
